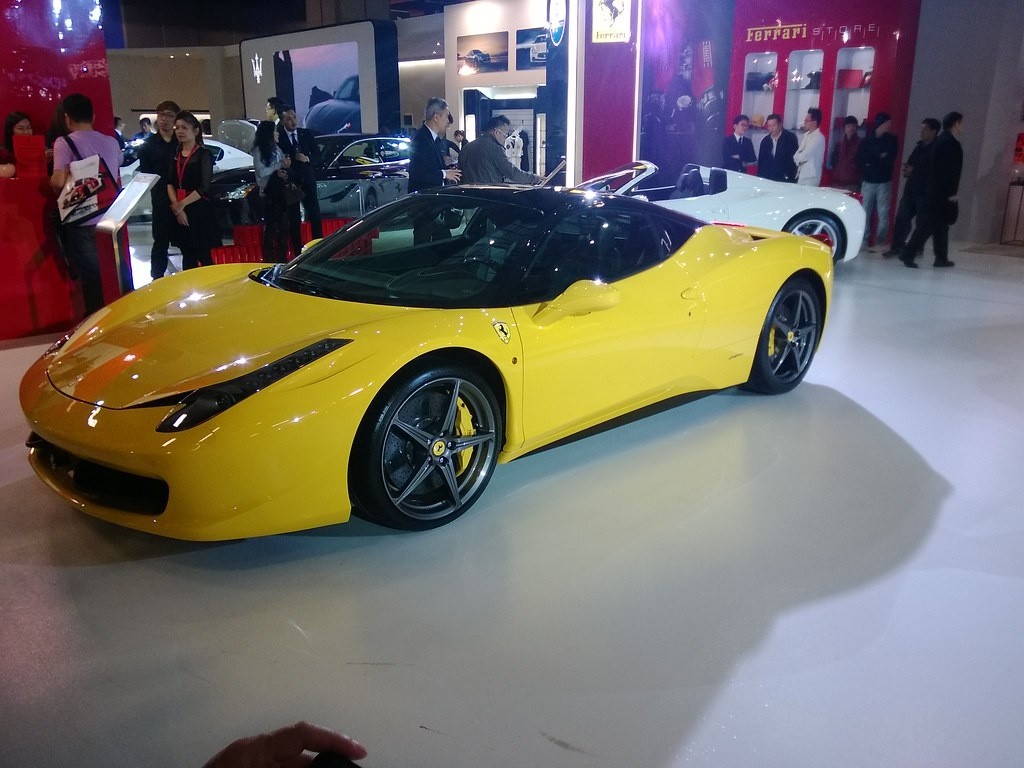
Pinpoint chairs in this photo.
[669,169,704,200]
[364,145,375,159]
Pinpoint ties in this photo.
[291,132,299,147]
[738,137,742,144]
[435,137,441,151]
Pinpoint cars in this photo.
[299,132,460,229]
[121,136,256,226]
[529,34,547,66]
[301,75,361,136]
[465,49,491,66]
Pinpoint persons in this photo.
[828,116,863,192]
[883,117,941,257]
[663,46,694,117]
[854,112,898,253]
[757,114,799,184]
[252,98,322,263]
[0,112,52,179]
[722,115,756,174]
[793,107,826,186]
[115,98,214,280]
[52,92,124,315]
[899,110,963,268]
[406,96,548,247]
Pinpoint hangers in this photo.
[509,123,522,137]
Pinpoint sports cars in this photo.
[465,162,867,287]
[18,182,831,543]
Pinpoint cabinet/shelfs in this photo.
[831,86,870,132]
[785,88,820,134]
[743,90,773,135]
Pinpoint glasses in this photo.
[157,110,176,118]
[495,128,508,139]
[805,119,813,122]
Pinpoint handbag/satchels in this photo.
[286,181,305,205]
[783,168,797,182]
[56,135,120,228]
[947,200,957,225]
[264,170,291,196]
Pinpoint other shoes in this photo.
[867,245,886,251]
[933,260,954,266]
[899,253,918,268]
[882,248,906,256]
[860,244,867,250]
